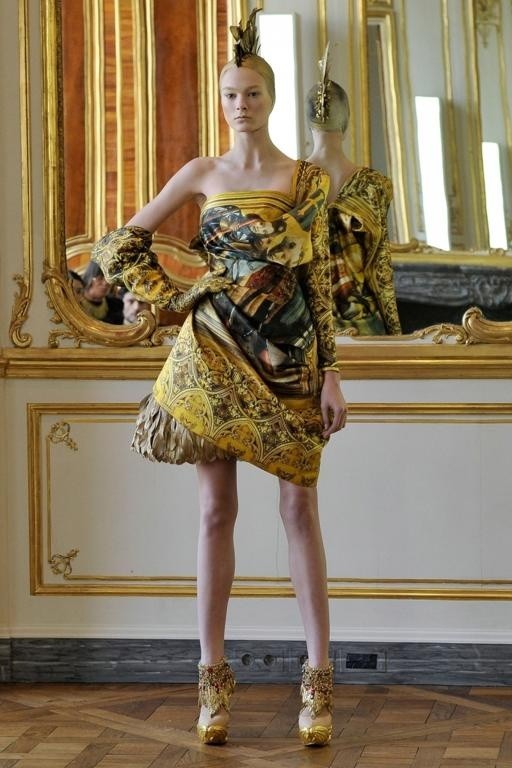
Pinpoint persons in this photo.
[79,257,125,328]
[68,268,85,304]
[90,54,348,750]
[304,78,403,336]
[116,286,153,328]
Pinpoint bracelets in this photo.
[321,366,340,372]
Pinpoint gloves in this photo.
[89,225,234,314]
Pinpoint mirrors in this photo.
[38,0,512,348]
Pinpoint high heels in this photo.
[297,658,335,747]
[196,657,236,745]
